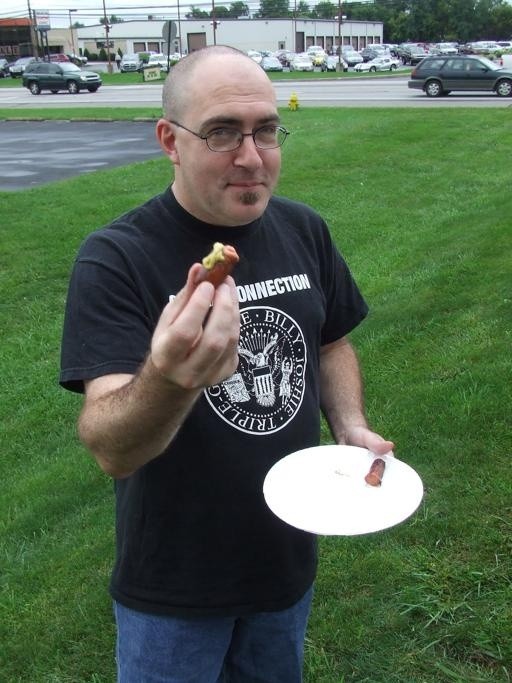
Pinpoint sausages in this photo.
[194,245,240,289]
[365,458,385,487]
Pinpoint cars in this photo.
[245,40,512,73]
[119,50,188,75]
[0,54,88,80]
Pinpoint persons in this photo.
[57,44,399,680]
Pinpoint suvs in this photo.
[408,54,512,97]
[22,62,103,95]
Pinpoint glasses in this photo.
[166,119,290,152]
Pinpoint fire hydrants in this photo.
[287,89,300,111]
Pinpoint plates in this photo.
[262,445,424,536]
